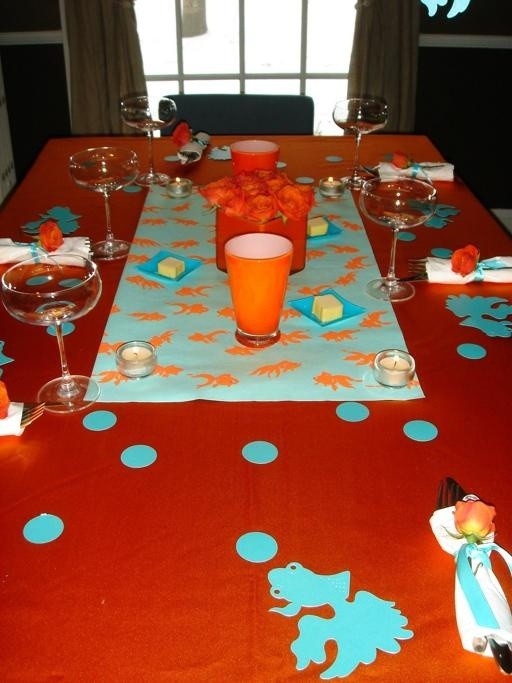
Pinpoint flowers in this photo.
[388,149,443,170]
[452,242,510,277]
[2,218,64,250]
[447,491,499,570]
[168,120,207,145]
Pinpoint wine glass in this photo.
[359,179,438,301]
[119,95,177,186]
[332,98,389,190]
[1,252,103,414]
[67,146,140,262]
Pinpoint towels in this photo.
[420,490,512,662]
[1,236,91,267]
[176,131,211,164]
[378,162,455,182]
[424,252,510,284]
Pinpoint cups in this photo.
[374,349,416,388]
[116,341,155,378]
[231,140,280,175]
[167,177,192,198]
[223,233,293,348]
[319,178,344,198]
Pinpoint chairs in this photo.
[157,93,317,135]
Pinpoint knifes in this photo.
[397,274,428,282]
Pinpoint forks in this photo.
[435,479,512,676]
[406,257,428,273]
[20,402,46,428]
